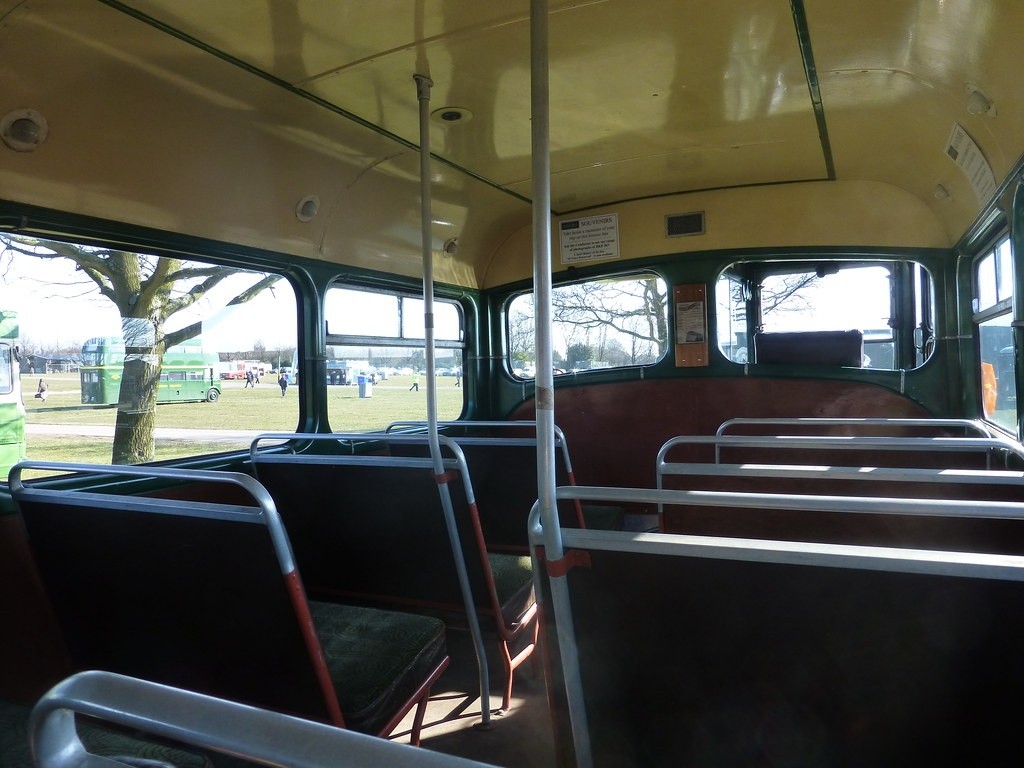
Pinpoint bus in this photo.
[0,310,27,479]
[77,336,222,410]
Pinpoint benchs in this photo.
[384,418,626,570]
[0,702,213,766]
[10,460,450,752]
[248,430,545,716]
[527,414,1024,768]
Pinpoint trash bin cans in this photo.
[358,375,373,399]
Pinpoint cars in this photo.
[366,365,463,376]
[510,360,567,380]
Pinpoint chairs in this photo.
[754,328,865,367]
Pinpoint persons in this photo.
[244,369,260,389]
[330,370,343,385]
[454,371,461,387]
[279,375,287,397]
[38,378,49,403]
[409,372,419,392]
